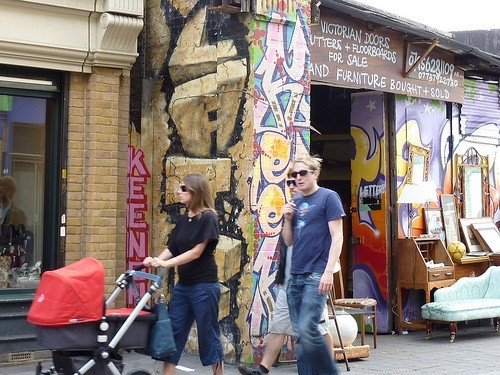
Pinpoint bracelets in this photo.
[284,218,288,222]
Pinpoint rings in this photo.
[154,263,155,264]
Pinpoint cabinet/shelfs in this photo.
[397,236,500,339]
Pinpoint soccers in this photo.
[448,241,466,259]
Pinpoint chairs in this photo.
[331,257,377,350]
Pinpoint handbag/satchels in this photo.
[127,270,176,359]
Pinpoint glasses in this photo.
[182,186,187,192]
[291,170,314,178]
[287,180,296,187]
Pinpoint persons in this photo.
[238,168,334,375]
[143,175,225,375]
[281,153,346,375]
[0,176,27,259]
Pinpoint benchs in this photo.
[421,265,500,344]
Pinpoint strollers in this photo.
[26,257,168,375]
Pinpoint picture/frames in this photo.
[476,226,500,253]
[457,216,494,253]
[469,222,500,252]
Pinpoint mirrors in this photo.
[440,194,460,247]
[455,146,490,218]
[422,208,446,248]
[405,144,430,186]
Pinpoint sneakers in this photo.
[238,364,268,375]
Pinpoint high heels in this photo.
[12,263,28,282]
[27,261,42,280]
